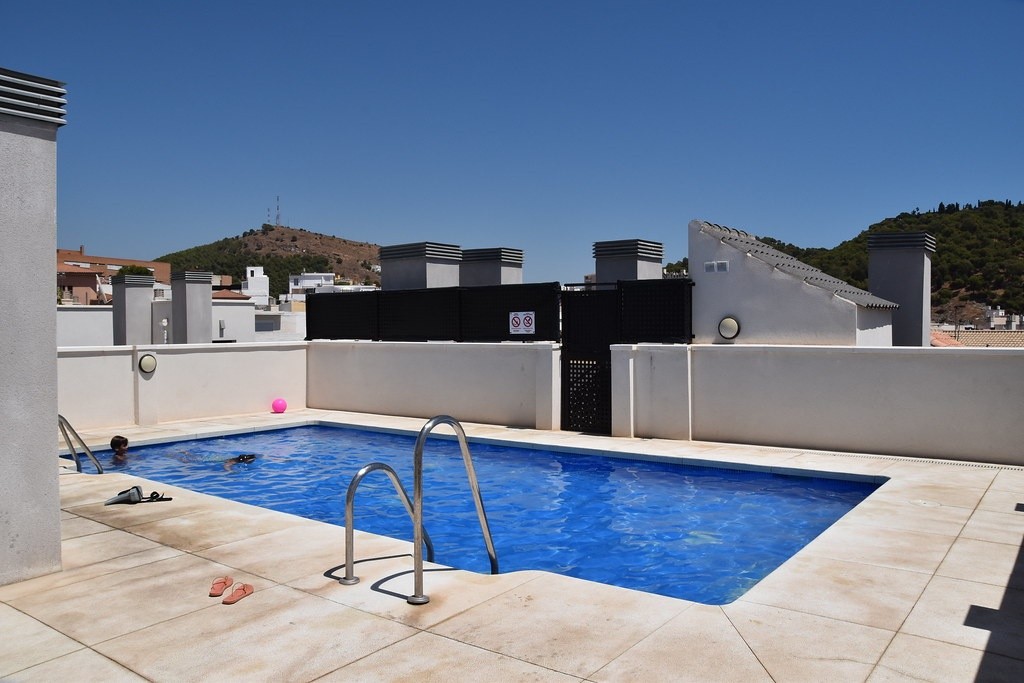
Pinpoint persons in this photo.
[167,448,257,476]
[109,435,129,467]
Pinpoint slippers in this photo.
[209,575,233,596]
[222,582,254,605]
